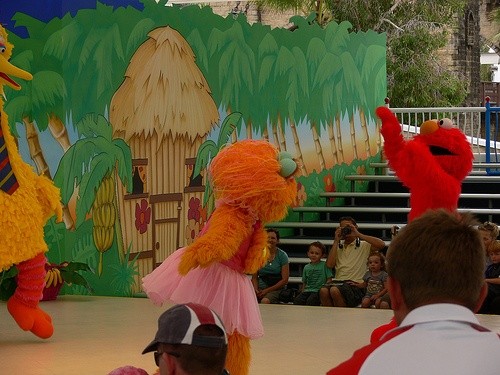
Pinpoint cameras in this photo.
[341,227,351,235]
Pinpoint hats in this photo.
[141,303,228,356]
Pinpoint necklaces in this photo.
[268,255,276,266]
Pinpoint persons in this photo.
[477,240,500,315]
[292,241,333,306]
[346,251,389,309]
[475,222,500,274]
[141,303,231,375]
[320,217,385,307]
[325,208,500,375]
[252,229,289,305]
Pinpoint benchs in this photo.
[264,164,500,284]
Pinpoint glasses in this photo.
[153,351,180,368]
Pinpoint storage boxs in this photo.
[40,280,62,302]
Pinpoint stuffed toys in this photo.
[138,139,299,375]
[369,106,474,344]
[1,24,63,338]
